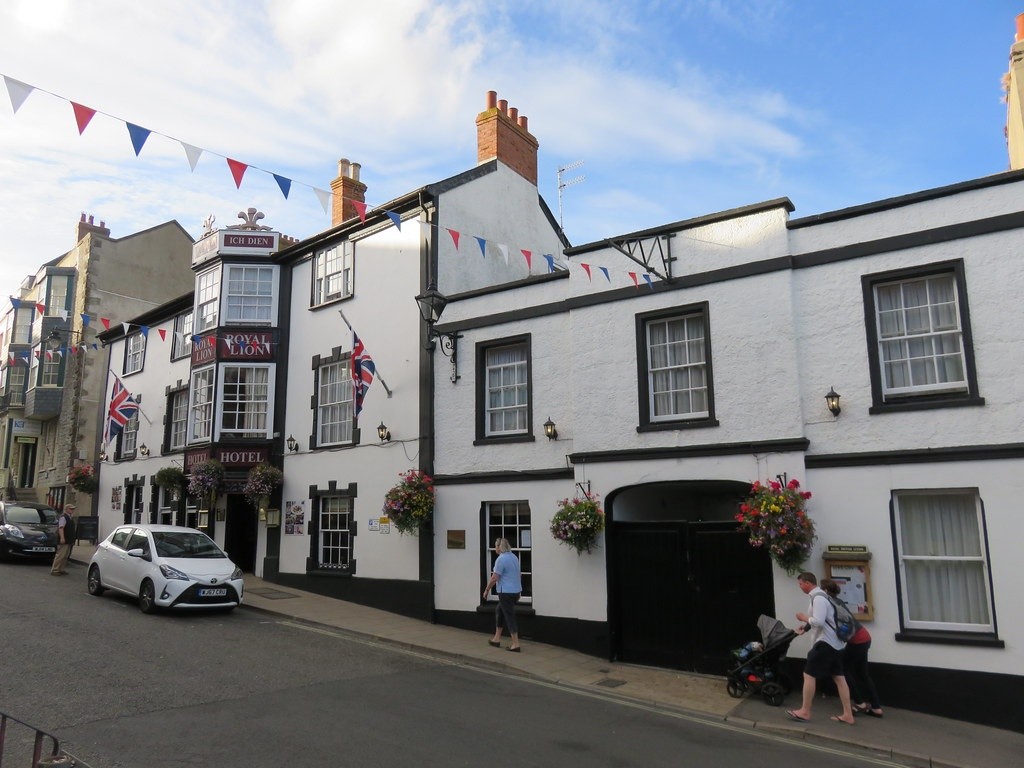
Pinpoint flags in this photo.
[104,377,139,446]
[351,329,375,417]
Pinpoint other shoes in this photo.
[61,571,68,575]
[505,647,520,652]
[488,639,500,647]
[50,571,62,576]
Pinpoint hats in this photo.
[65,504,77,510]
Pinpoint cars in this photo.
[86,524,245,613]
[0,501,61,562]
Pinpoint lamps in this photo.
[543,416,559,442]
[140,442,150,456]
[99,449,108,462]
[47,325,81,348]
[286,434,299,453]
[377,421,391,441]
[824,386,841,416]
[415,278,464,385]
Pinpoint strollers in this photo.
[717,613,799,707]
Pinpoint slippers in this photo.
[831,715,854,725]
[863,708,882,717]
[850,705,867,713]
[785,709,808,722]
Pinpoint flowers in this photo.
[549,491,606,554]
[186,460,224,497]
[734,479,818,577]
[242,463,283,501]
[67,462,98,494]
[381,471,438,533]
[155,467,184,499]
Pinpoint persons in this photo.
[483,538,522,652]
[739,642,764,672]
[785,572,854,725]
[820,579,884,718]
[51,504,78,576]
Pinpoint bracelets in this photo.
[485,589,489,591]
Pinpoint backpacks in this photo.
[810,593,854,642]
